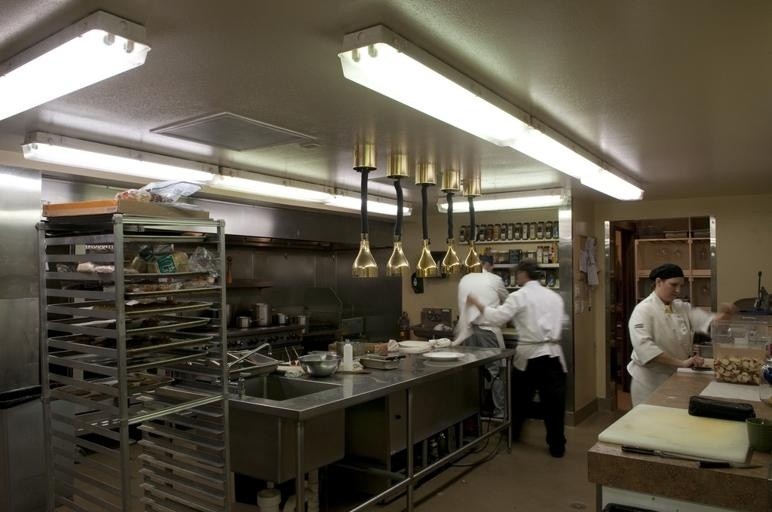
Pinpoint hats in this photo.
[648,263,685,285]
[477,254,495,264]
[512,259,544,276]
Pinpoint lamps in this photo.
[347,141,484,280]
[0,9,153,125]
[20,131,414,219]
[437,190,569,217]
[339,26,644,204]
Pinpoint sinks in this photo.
[218,375,346,486]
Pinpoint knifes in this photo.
[698,459,765,470]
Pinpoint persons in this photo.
[467,259,568,458]
[453,256,509,436]
[626,264,736,409]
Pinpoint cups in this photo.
[227,302,307,328]
[746,416,771,453]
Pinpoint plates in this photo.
[394,336,466,362]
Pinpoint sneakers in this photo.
[499,427,521,443]
[548,442,570,458]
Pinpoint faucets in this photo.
[226,342,273,382]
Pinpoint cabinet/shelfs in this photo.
[457,238,560,292]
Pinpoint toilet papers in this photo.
[343,374,353,398]
[343,344,353,371]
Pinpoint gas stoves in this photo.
[206,329,310,351]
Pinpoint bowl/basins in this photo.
[295,348,338,378]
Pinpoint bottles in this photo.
[458,221,559,242]
[340,342,355,373]
[758,342,772,406]
[485,245,559,263]
[492,267,560,288]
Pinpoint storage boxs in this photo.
[709,320,769,385]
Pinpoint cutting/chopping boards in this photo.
[596,403,756,463]
[676,355,715,378]
